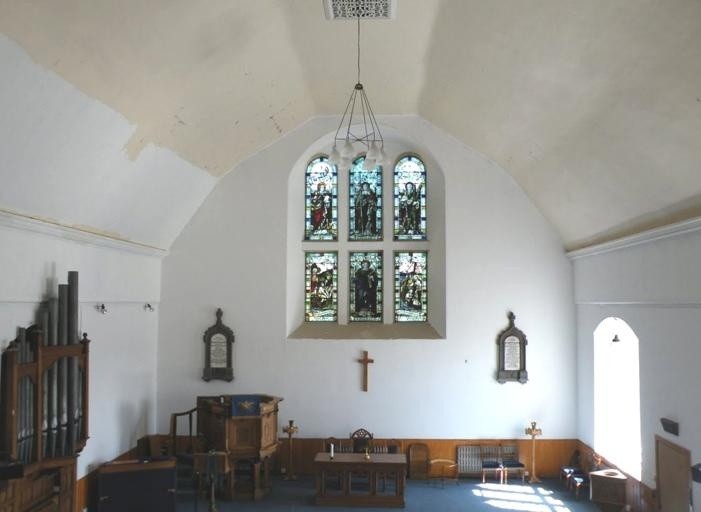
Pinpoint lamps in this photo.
[95,304,107,313]
[328,1,392,171]
[144,303,154,312]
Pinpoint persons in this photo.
[353,259,378,317]
[350,180,381,234]
[398,180,424,234]
[304,181,336,233]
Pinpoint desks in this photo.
[96,458,176,512]
[313,452,407,508]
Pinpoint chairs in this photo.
[456,443,526,486]
[559,452,586,490]
[326,429,407,493]
[570,454,601,500]
